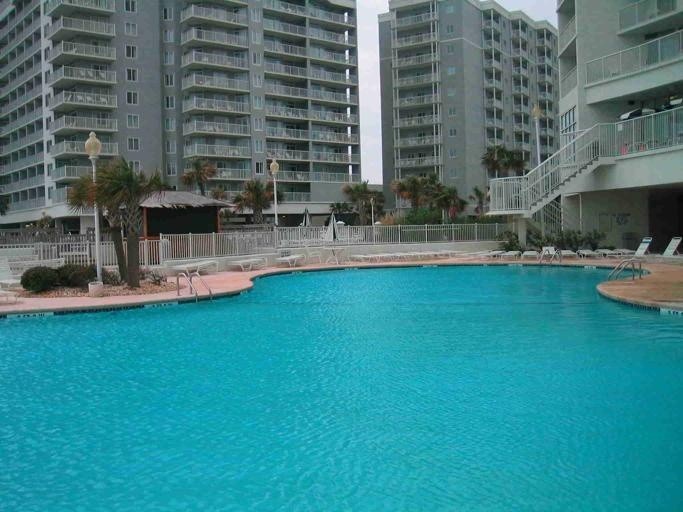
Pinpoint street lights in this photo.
[270,158,279,225]
[370,198,375,226]
[85,132,102,283]
[531,103,546,247]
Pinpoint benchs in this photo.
[164,234,681,273]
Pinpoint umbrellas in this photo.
[322,212,340,248]
[301,206,310,227]
[660,97,683,137]
[615,106,666,142]
